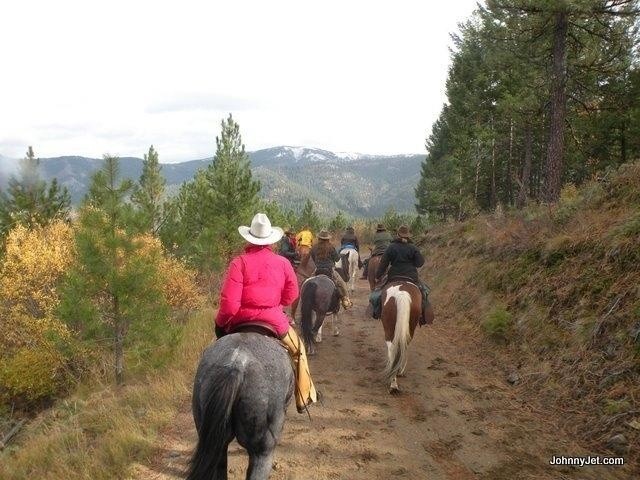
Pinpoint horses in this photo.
[183,333,295,480]
[378,281,422,393]
[290,245,317,326]
[340,248,359,299]
[300,252,350,356]
[367,246,384,293]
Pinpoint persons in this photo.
[340,227,362,269]
[213,211,325,416]
[279,228,299,269]
[359,224,394,280]
[310,229,353,310]
[369,225,433,325]
[295,225,312,249]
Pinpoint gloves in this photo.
[215,322,224,338]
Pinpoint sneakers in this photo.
[345,302,353,310]
[297,391,322,413]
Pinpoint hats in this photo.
[238,213,296,246]
[316,229,332,240]
[376,224,386,230]
[397,224,413,239]
